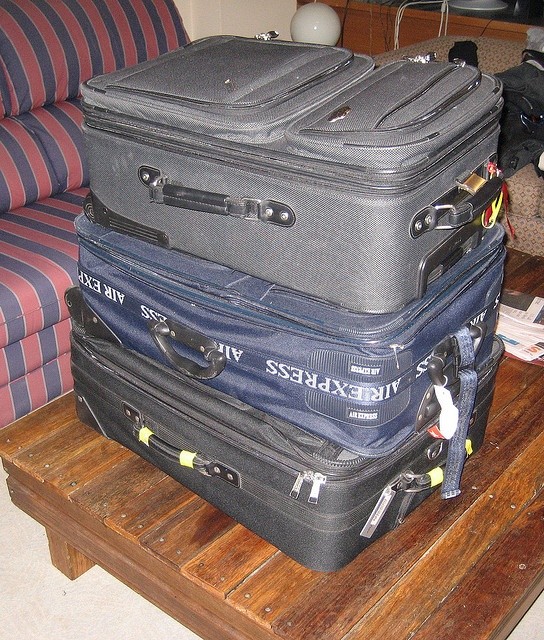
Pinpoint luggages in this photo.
[73,208,510,502]
[77,32,508,316]
[69,323,507,576]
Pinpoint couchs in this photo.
[1,2,194,430]
[372,35,544,259]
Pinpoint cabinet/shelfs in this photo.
[296,0,544,58]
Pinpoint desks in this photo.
[0,250,544,640]
